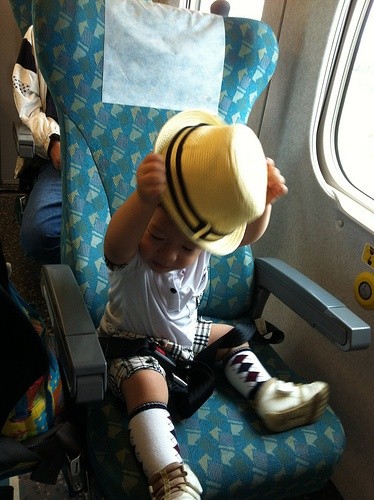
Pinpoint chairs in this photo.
[11,1,372,500]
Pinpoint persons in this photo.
[97,153,328,500]
[12,24,63,264]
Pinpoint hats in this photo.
[153,108,267,256]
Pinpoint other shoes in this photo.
[250,376,329,433]
[147,462,202,500]
